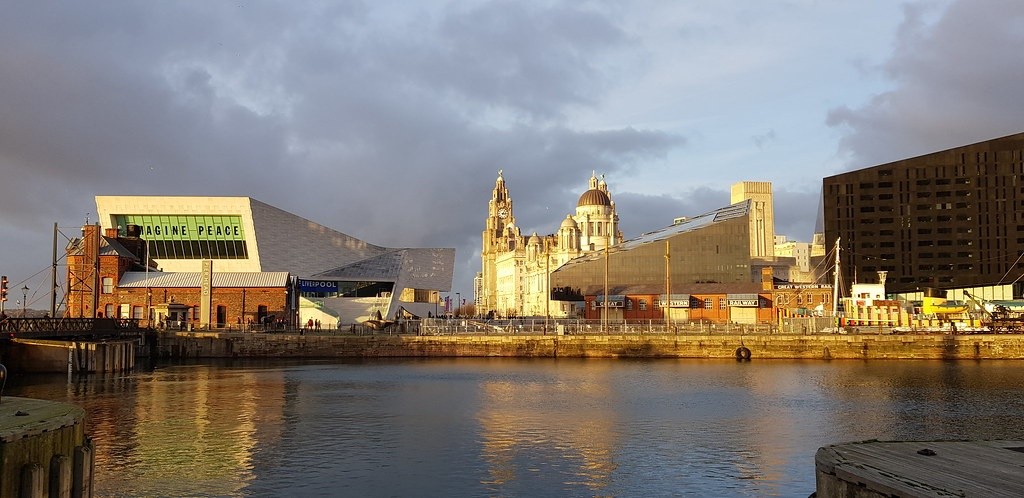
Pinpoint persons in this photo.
[44,313,50,318]
[237,316,241,331]
[318,320,322,332]
[164,315,169,327]
[428,311,432,318]
[314,319,318,332]
[178,315,184,332]
[0,311,7,320]
[308,319,313,331]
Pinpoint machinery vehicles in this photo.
[962,290,1023,332]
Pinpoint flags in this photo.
[445,296,450,311]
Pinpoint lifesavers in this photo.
[737,347,751,359]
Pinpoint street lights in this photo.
[21,285,29,316]
